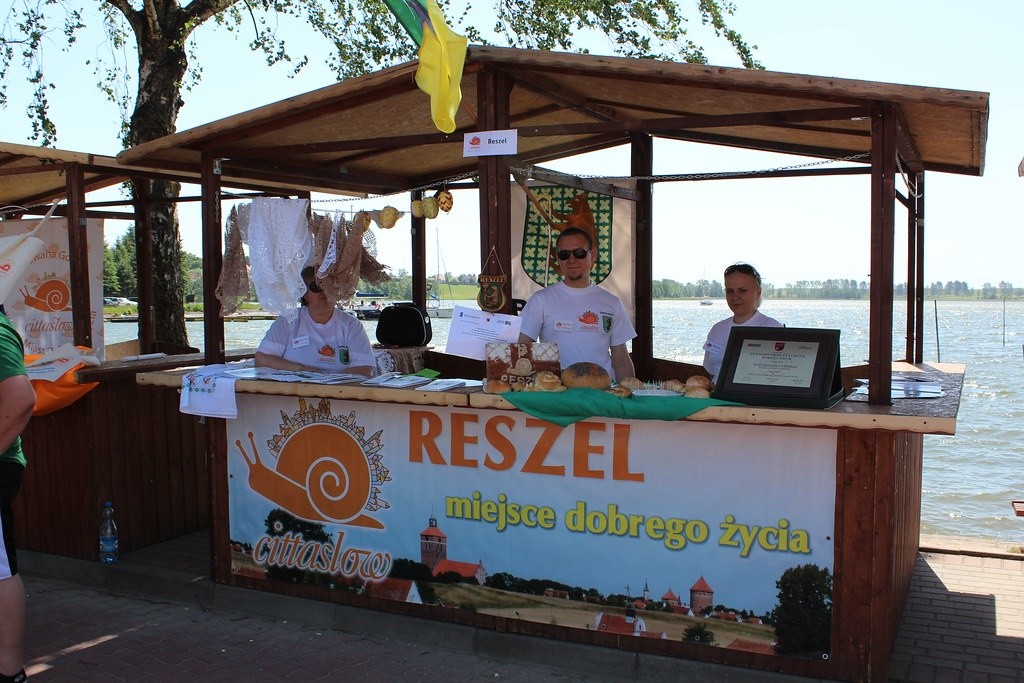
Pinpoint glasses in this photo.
[725,264,760,285]
[555,248,591,260]
[309,282,323,293]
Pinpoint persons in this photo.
[374,303,380,310]
[703,264,784,386]
[0,310,37,683]
[518,227,637,383]
[255,266,374,378]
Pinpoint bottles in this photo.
[98,502,119,564]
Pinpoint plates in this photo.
[633,391,685,397]
[611,382,659,390]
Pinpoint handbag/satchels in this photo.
[486,342,561,383]
[477,246,508,312]
[375,302,432,346]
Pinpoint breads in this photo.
[485,361,715,399]
